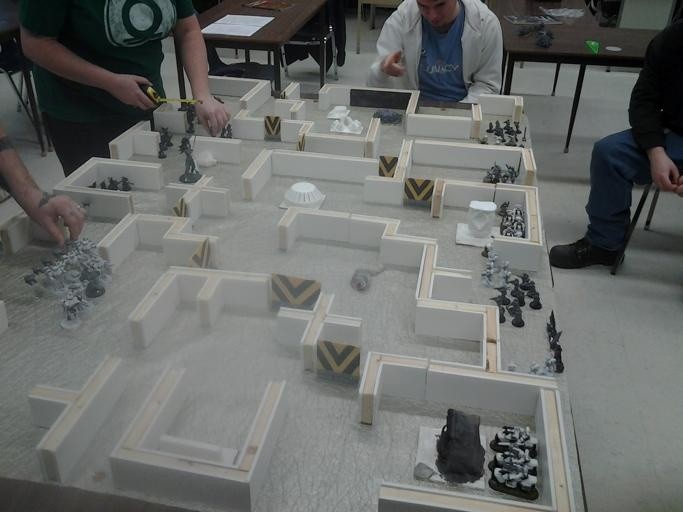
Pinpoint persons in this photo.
[549,1,682,269]
[0,119,86,249]
[366,0,503,104]
[17,0,231,179]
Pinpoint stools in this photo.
[357,0,404,54]
[281,23,338,92]
[610,170,683,275]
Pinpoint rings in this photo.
[76,205,80,208]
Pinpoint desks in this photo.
[168,1,329,112]
[483,1,665,153]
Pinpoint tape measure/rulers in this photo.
[140,85,203,104]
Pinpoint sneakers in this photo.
[550,238,625,269]
